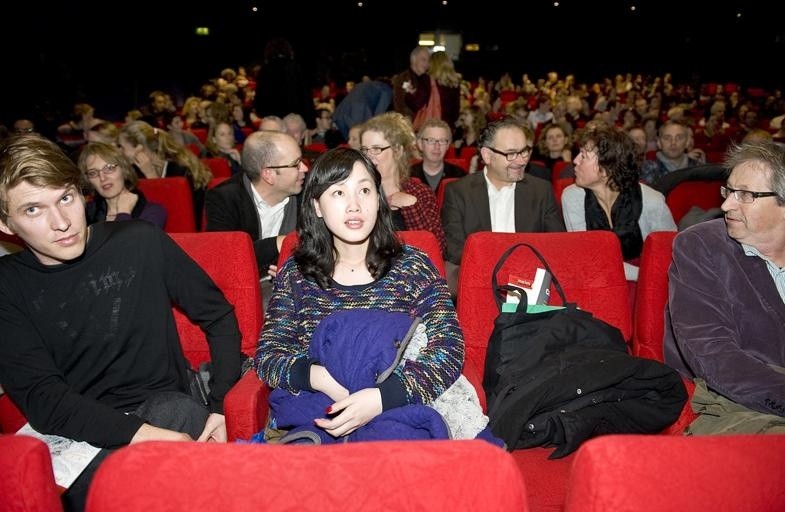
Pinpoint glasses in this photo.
[480,145,531,161]
[422,137,449,145]
[359,145,392,155]
[264,159,302,168]
[85,163,119,178]
[720,185,778,203]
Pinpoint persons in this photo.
[0,133,242,510]
[253,145,467,447]
[0,44,785,316]
[662,138,784,434]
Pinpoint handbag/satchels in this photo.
[413,73,442,132]
[481,242,628,415]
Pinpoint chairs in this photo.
[274,230,448,288]
[635,230,699,433]
[566,433,784,509]
[165,232,272,443]
[81,439,531,512]
[456,227,635,422]
[0,435,67,511]
[1,88,785,232]
